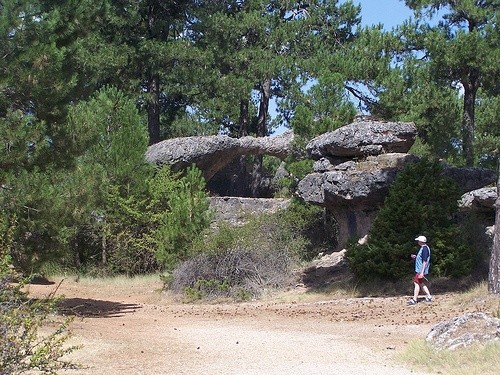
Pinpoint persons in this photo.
[408,235,433,305]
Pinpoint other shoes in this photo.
[424,297,433,303]
[410,298,418,305]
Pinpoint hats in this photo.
[414,236,427,242]
